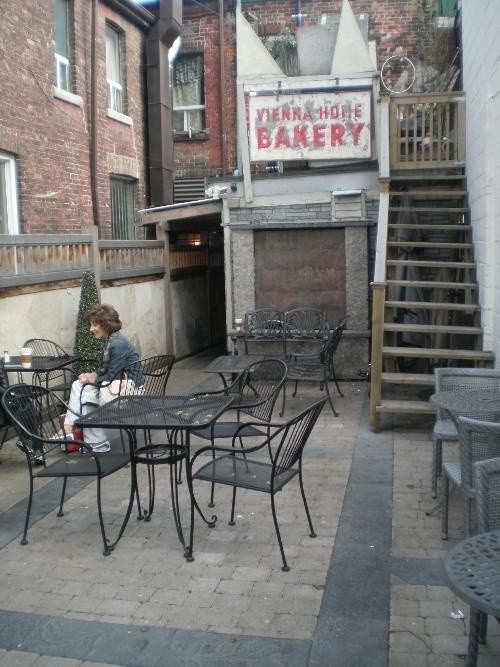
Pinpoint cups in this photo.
[19,348,33,369]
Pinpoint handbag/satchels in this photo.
[99,372,135,409]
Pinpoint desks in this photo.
[443,529,500,667]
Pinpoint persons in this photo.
[0,360,45,465]
[48,303,145,454]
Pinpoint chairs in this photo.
[431,368,500,646]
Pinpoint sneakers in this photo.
[16,437,44,464]
[49,434,74,441]
[79,440,111,454]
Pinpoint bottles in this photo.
[3,350,11,364]
